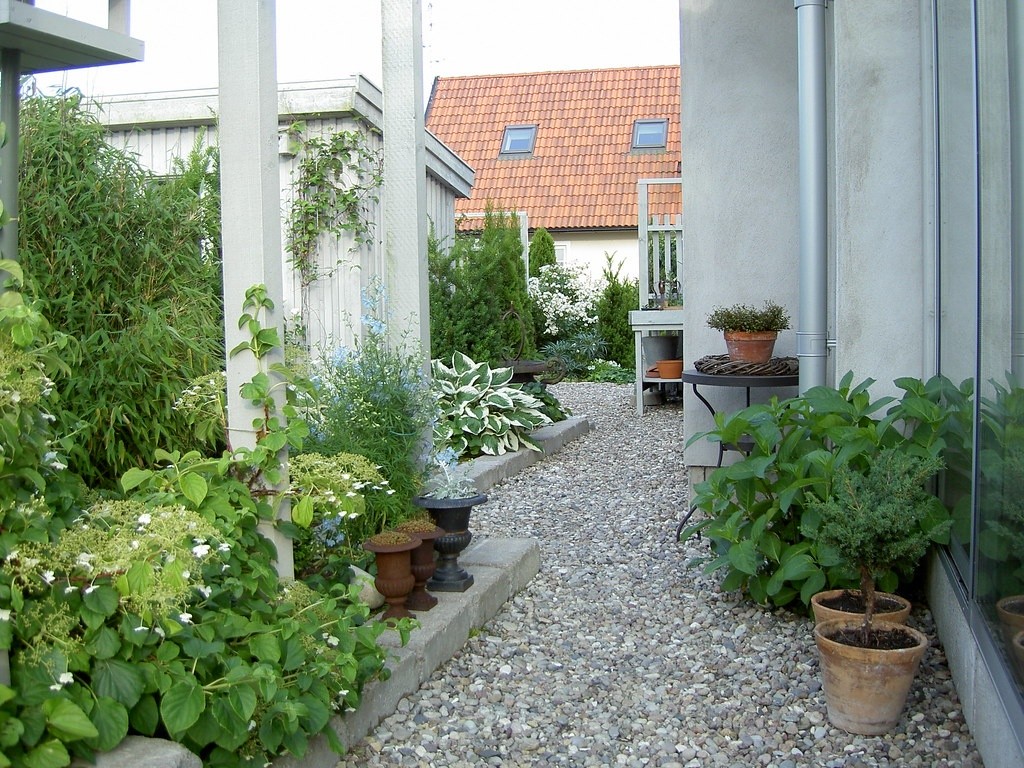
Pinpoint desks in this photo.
[676,369,799,542]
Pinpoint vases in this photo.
[656,360,683,378]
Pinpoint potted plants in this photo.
[702,297,793,364]
[393,518,446,611]
[365,529,422,627]
[813,465,956,736]
[996,466,1024,688]
[412,456,490,592]
[983,443,1024,680]
[792,435,950,630]
